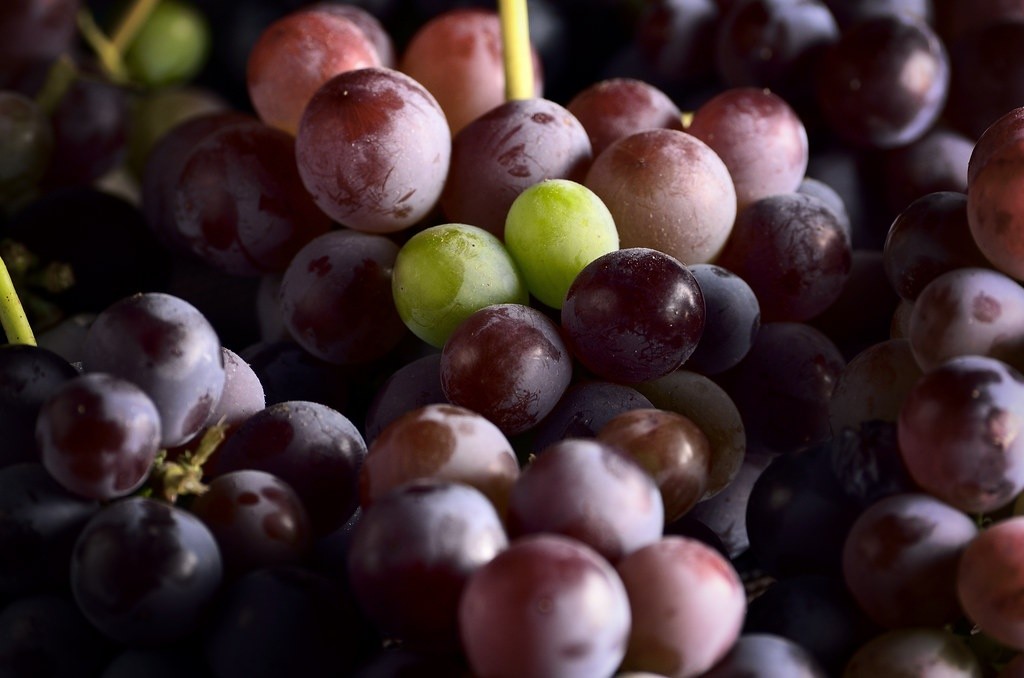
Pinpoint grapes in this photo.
[0,0,1024,678]
